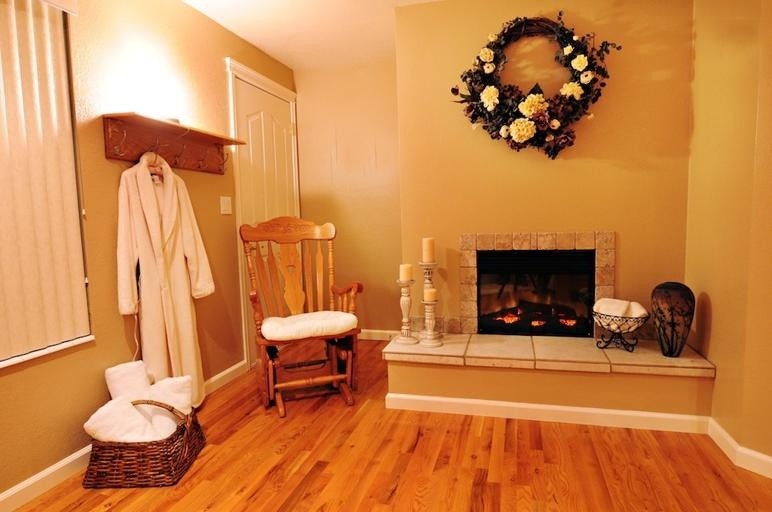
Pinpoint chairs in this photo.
[238,217,364,419]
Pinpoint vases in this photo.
[650,282,696,358]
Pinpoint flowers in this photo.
[448,15,623,161]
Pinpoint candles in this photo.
[399,263,412,282]
[422,238,436,263]
[423,288,437,302]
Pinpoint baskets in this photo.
[81,399,207,489]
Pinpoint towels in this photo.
[83,359,194,443]
